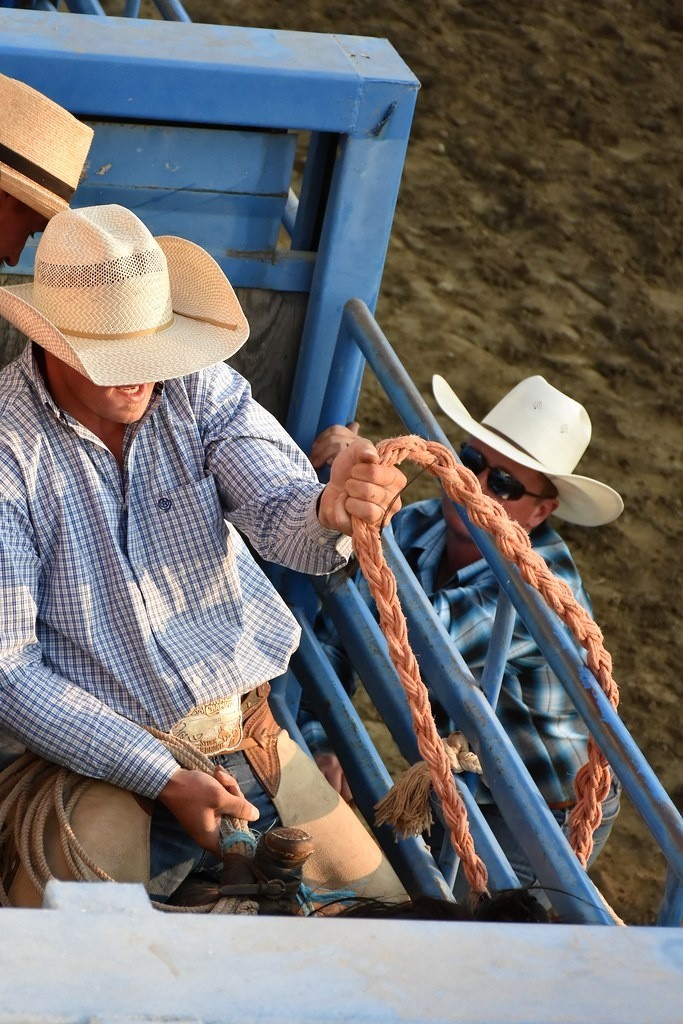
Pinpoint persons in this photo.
[0,202,408,908]
[0,72,96,273]
[309,375,622,910]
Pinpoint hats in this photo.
[0,201,250,387]
[430,373,625,528]
[0,69,97,218]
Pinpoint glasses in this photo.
[456,440,556,505]
